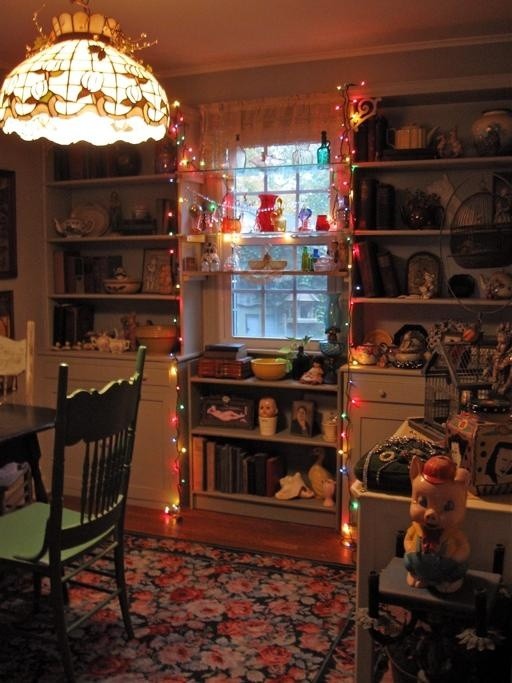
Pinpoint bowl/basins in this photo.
[134,326,177,353]
[252,358,289,381]
[103,279,143,294]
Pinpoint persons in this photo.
[298,356,324,384]
[293,407,309,436]
[259,398,278,418]
[208,406,245,421]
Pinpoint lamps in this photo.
[0,12,170,146]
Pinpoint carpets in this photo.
[1,531,426,683]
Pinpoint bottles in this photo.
[301,246,309,271]
[228,248,239,271]
[309,248,319,271]
[202,259,209,272]
[315,130,330,163]
[292,142,313,165]
[274,208,286,232]
[227,133,245,167]
[211,260,219,272]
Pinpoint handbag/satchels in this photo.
[354,436,447,496]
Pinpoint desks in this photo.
[0,404,57,516]
[355,489,512,683]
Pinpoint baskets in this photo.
[450,192,512,269]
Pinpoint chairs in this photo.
[0,321,32,407]
[0,347,146,683]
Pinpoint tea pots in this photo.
[387,341,425,369]
[350,342,388,365]
[53,211,96,238]
[386,122,440,151]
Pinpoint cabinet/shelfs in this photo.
[338,76,512,545]
[188,357,341,528]
[37,173,206,511]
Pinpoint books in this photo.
[192,437,284,496]
[198,342,253,378]
